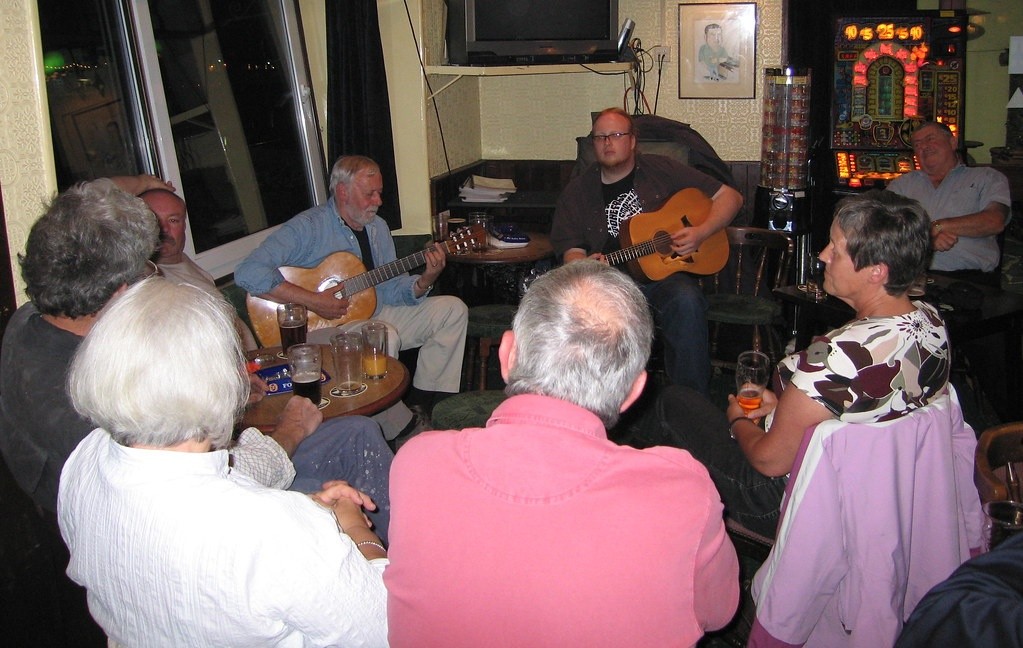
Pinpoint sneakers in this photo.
[396,405,434,442]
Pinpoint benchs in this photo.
[0,235,434,648]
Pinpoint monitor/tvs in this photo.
[445,0,620,66]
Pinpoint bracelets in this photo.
[934,221,943,232]
[357,541,386,552]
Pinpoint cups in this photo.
[287,342,322,406]
[736,351,771,426]
[276,302,309,358]
[361,321,389,381]
[469,211,491,254]
[447,218,466,238]
[432,210,450,244]
[329,331,363,393]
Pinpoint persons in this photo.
[233,158,468,440]
[0,176,391,648]
[626,188,950,561]
[550,107,743,396]
[382,257,740,648]
[883,120,1013,401]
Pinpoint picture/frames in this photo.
[71,98,133,178]
[678,2,757,100]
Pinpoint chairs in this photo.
[574,114,740,373]
[973,421,1023,554]
[698,227,795,397]
[724,382,993,648]
[969,164,1023,295]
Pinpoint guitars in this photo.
[246,222,488,349]
[518,187,731,297]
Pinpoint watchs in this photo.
[728,417,754,440]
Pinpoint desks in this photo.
[446,229,553,305]
[234,345,411,436]
[449,193,562,216]
[771,271,1023,422]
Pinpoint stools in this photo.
[432,391,509,431]
[465,305,520,392]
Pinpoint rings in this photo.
[432,264,437,267]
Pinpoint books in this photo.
[458,167,521,204]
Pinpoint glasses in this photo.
[591,130,633,142]
[145,259,159,279]
